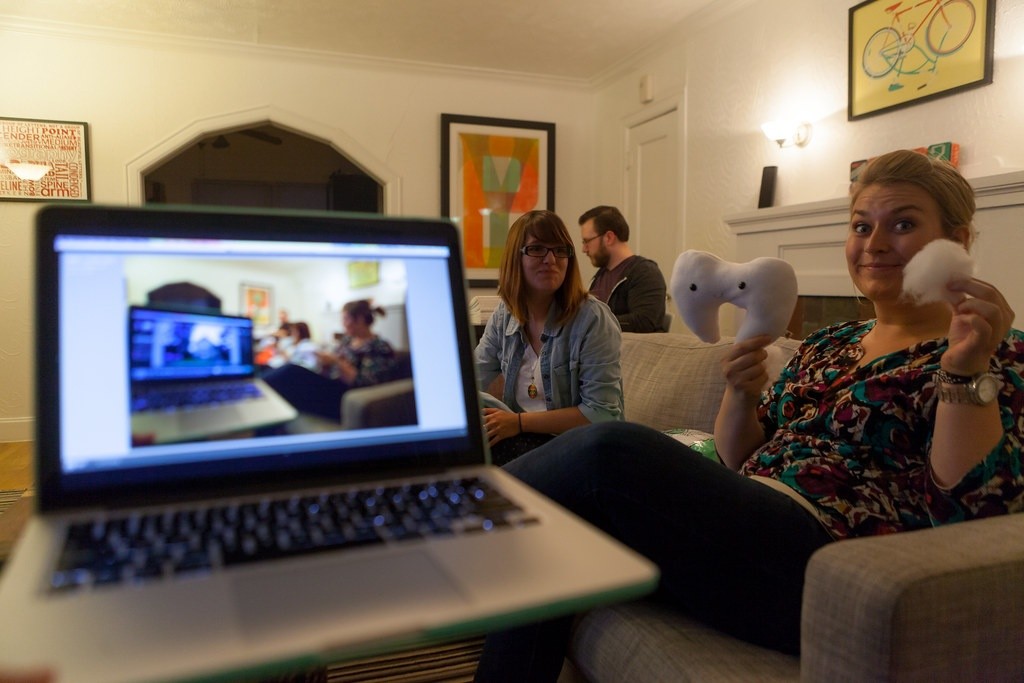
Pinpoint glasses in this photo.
[583,232,606,243]
[522,245,574,258]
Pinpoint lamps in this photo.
[760,119,812,148]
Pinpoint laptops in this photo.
[0,203,660,683]
[127,305,299,448]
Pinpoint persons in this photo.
[254,300,395,437]
[579,205,666,334]
[471,150,1024,682]
[473,210,622,467]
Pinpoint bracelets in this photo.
[937,369,984,385]
[519,412,523,433]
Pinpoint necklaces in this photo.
[519,330,545,399]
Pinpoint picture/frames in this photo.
[847,0,995,122]
[0,116,91,204]
[439,113,557,289]
[347,261,379,288]
[239,281,275,327]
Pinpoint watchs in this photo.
[942,373,999,405]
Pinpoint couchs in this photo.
[571,329,1024,683]
[281,349,414,436]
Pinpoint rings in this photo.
[483,425,488,432]
